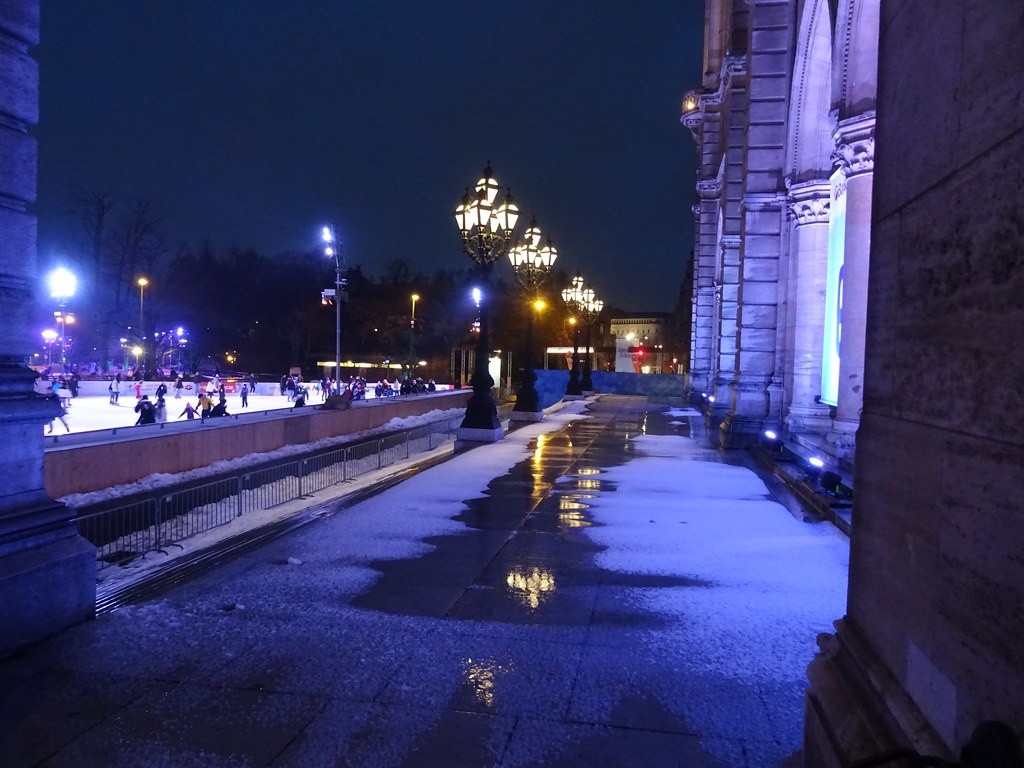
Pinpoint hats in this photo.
[251,374,254,376]
[243,384,246,386]
[198,394,203,398]
[178,374,183,378]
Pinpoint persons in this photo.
[109,373,120,406]
[250,373,257,394]
[33,367,41,388]
[239,384,249,407]
[293,394,306,407]
[46,385,69,434]
[195,394,215,418]
[313,376,368,402]
[52,374,79,397]
[133,380,143,399]
[135,395,156,425]
[208,399,230,417]
[173,375,184,398]
[280,374,309,402]
[218,384,226,403]
[154,398,167,422]
[178,402,201,419]
[156,384,168,400]
[190,371,219,398]
[375,375,436,397]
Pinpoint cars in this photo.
[268,369,287,377]
[389,375,408,383]
[334,380,349,388]
[102,370,127,381]
[311,378,324,383]
[132,365,163,380]
[88,372,100,381]
[291,374,303,382]
[231,372,252,379]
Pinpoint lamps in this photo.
[764,429,784,457]
[803,458,822,486]
[820,470,853,501]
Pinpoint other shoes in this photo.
[114,402,120,406]
[250,393,251,394]
[48,430,52,434]
[253,393,254,394]
[67,428,70,432]
[110,401,114,405]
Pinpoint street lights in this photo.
[560,271,595,401]
[506,214,558,422]
[138,279,146,321]
[57,275,68,371]
[321,224,348,396]
[580,283,605,395]
[452,158,523,442]
[135,348,140,366]
[410,295,418,364]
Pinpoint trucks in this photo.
[289,367,302,382]
